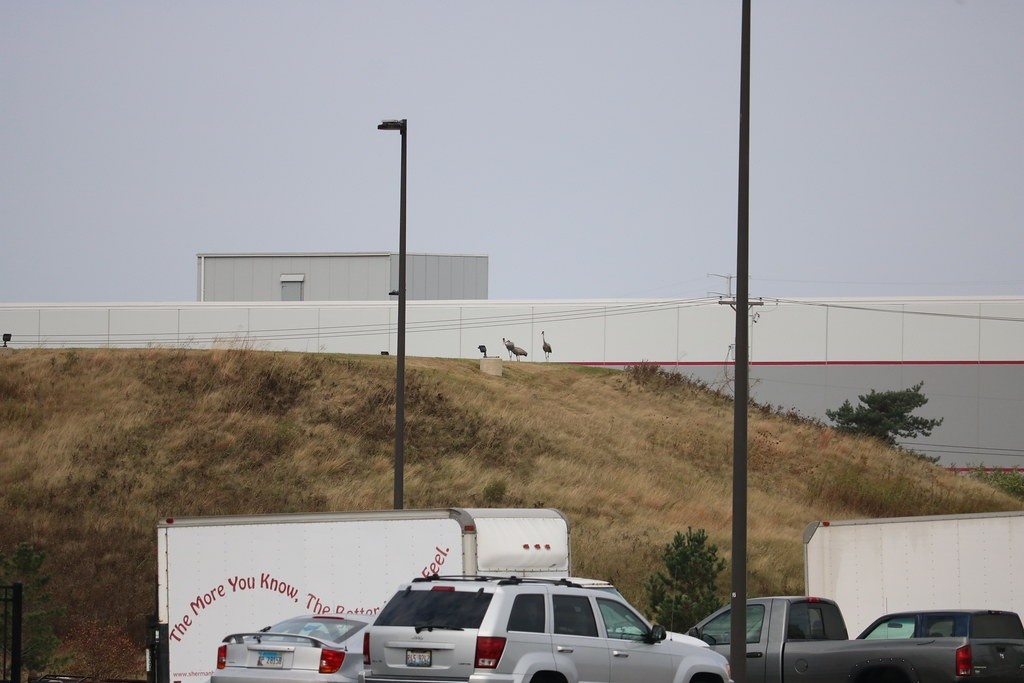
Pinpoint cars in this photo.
[211,610,380,683]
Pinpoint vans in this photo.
[852,608,1024,639]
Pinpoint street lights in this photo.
[378,119,409,510]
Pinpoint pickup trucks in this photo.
[680,595,1024,683]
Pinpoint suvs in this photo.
[358,572,737,683]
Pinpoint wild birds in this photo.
[541,330,552,360]
[502,337,528,363]
